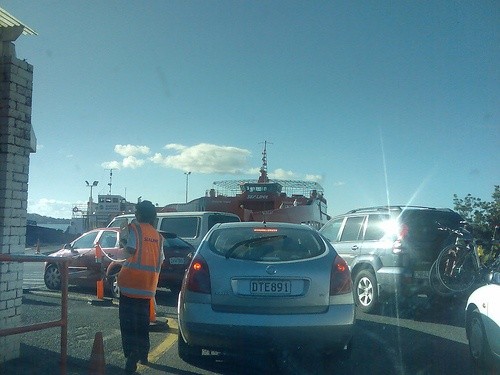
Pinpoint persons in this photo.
[117,200,162,375]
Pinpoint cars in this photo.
[465,269,500,367]
[43,228,196,297]
[176,220,355,356]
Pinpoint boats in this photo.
[160,142,328,226]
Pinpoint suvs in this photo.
[319,206,473,313]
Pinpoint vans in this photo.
[106,210,242,249]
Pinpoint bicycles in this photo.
[429,221,500,296]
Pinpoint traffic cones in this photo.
[86,332,108,375]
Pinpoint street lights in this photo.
[85,180,99,230]
[184,171,191,204]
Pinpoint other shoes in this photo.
[125,352,140,375]
[140,357,149,365]
